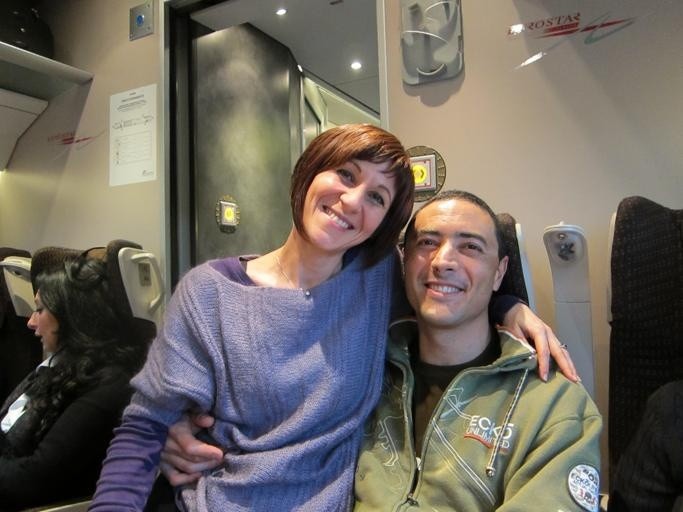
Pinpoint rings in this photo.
[561,343,568,350]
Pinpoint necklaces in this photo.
[267,246,302,297]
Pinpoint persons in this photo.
[0,255,174,512]
[606,378,683,511]
[159,189,603,512]
[86,123,584,512]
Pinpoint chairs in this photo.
[609,196,683,495]
[399,213,536,311]
[30,240,164,512]
[0,248,40,403]
